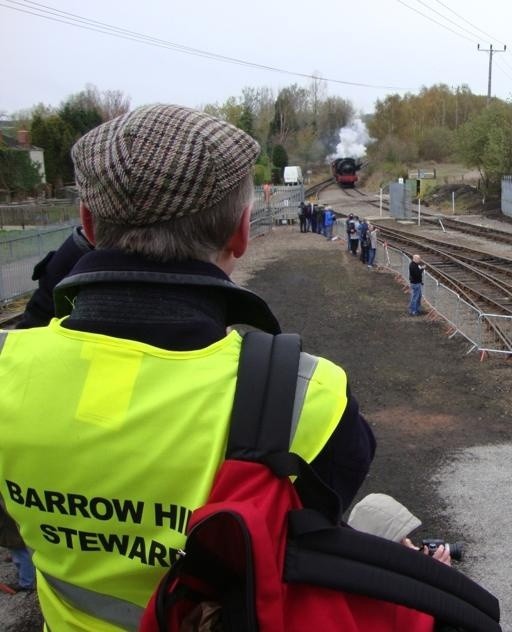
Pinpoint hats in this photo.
[69,102,261,227]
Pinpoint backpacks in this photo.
[138,330,504,631]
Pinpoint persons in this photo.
[343,492,453,569]
[408,254,426,316]
[261,182,273,205]
[0,100,378,631]
[296,200,380,270]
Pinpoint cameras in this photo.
[417,536,463,562]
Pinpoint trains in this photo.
[331,157,359,187]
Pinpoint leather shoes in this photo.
[6,582,33,594]
